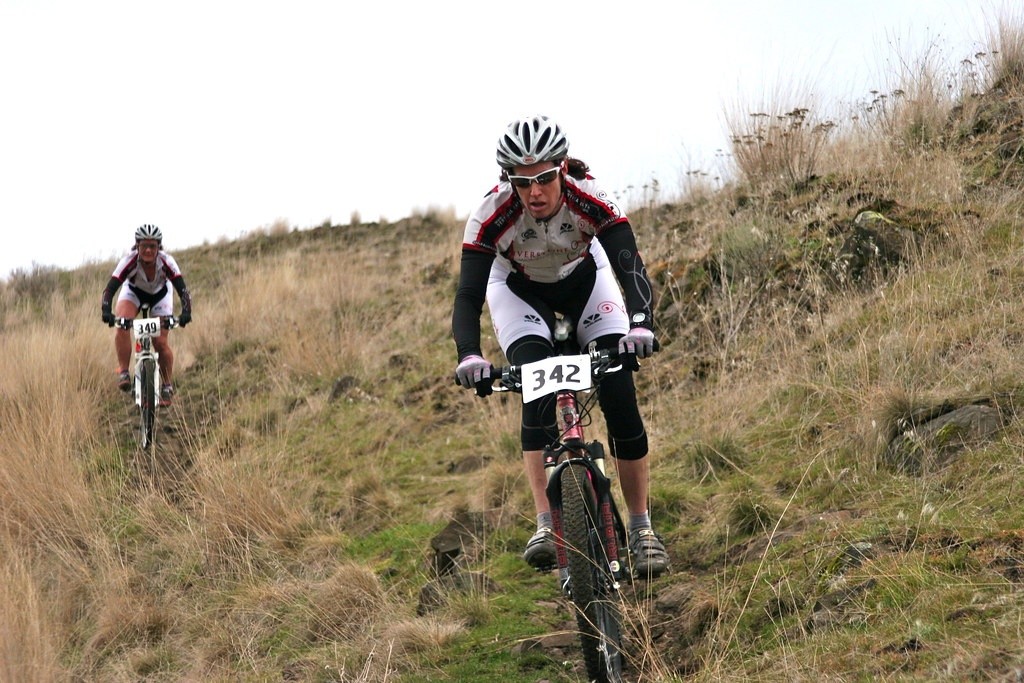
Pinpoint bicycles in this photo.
[454,333,664,680]
[106,315,181,450]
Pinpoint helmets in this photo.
[135,223,162,241]
[496,114,570,169]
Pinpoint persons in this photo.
[449,115,670,574]
[101,224,192,407]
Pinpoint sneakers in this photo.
[524,522,556,568]
[118,370,131,392]
[159,384,174,407]
[628,528,668,573]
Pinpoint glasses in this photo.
[506,160,564,187]
[137,243,159,249]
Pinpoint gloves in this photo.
[102,308,115,327]
[618,326,654,372]
[179,310,191,327]
[455,355,496,398]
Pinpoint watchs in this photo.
[632,313,650,323]
